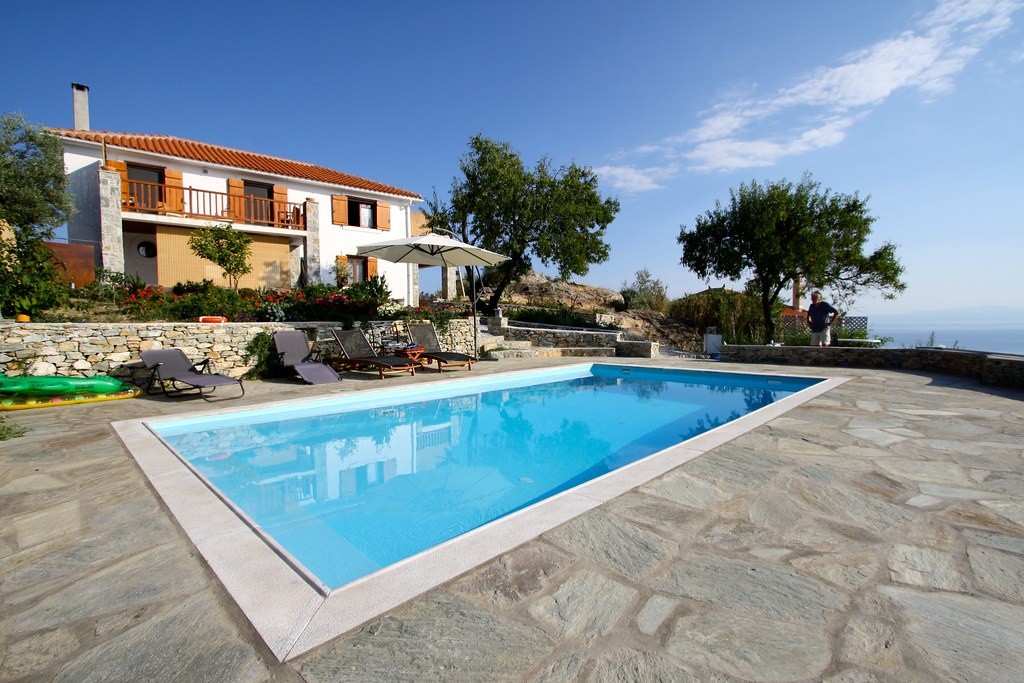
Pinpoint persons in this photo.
[807,291,838,347]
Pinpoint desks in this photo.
[837,338,881,347]
[393,347,425,377]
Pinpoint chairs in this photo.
[138,348,245,403]
[272,330,343,385]
[405,322,478,374]
[331,329,423,380]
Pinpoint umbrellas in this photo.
[355,232,512,267]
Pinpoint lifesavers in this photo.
[195,315,228,323]
